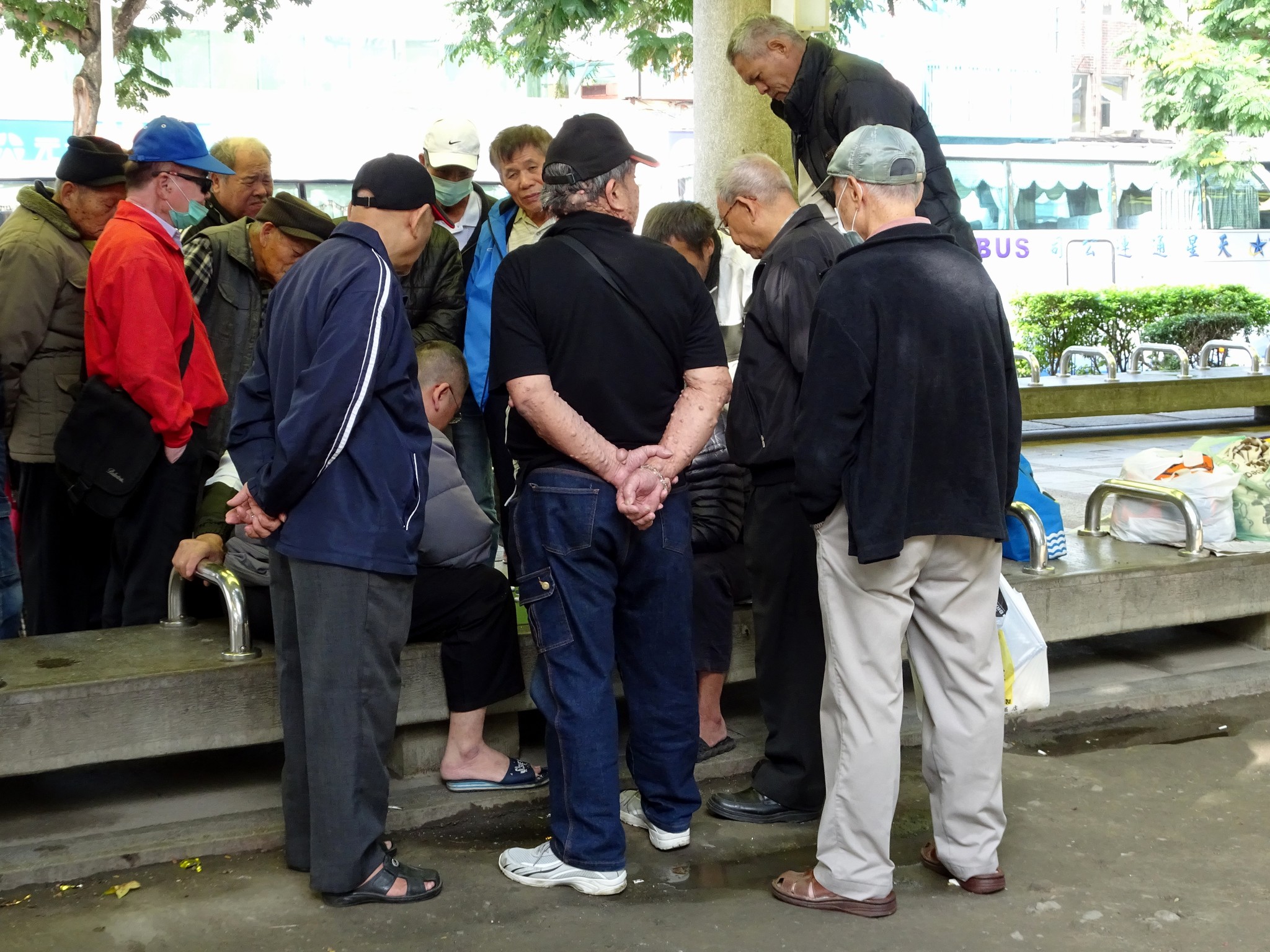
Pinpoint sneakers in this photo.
[498,839,628,896]
[619,789,691,852]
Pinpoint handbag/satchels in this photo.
[907,574,1050,724]
[51,375,164,519]
[1004,452,1067,560]
[1110,448,1239,549]
[1184,429,1270,540]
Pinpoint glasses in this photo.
[717,196,757,238]
[151,171,213,193]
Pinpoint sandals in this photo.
[322,857,444,908]
[292,833,397,873]
[771,870,897,917]
[921,841,1008,893]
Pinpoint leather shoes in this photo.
[712,759,823,824]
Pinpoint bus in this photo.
[932,129,1270,324]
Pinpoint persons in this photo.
[726,13,983,264]
[771,125,1023,916]
[0,113,854,908]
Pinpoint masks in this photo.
[833,183,865,247]
[426,166,472,206]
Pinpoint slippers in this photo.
[695,736,736,764]
[446,756,548,794]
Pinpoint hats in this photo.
[254,192,337,244]
[350,153,454,228]
[423,117,479,171]
[812,123,927,197]
[128,115,237,175]
[541,113,660,185]
[55,136,128,187]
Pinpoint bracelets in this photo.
[640,464,667,490]
